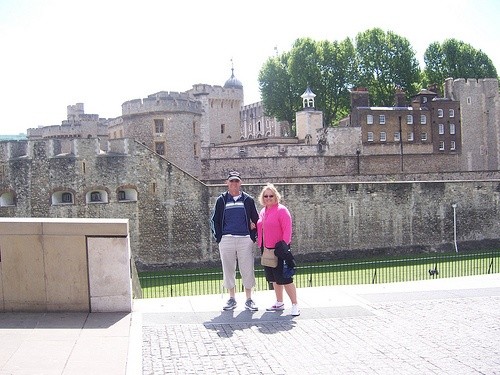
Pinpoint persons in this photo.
[250,184,302,316]
[210,171,260,311]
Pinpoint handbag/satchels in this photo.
[261,247,279,268]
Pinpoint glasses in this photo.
[230,179,240,183]
[264,195,274,198]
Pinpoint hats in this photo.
[227,171,242,181]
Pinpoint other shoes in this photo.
[266,302,284,311]
[292,304,300,315]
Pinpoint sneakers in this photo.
[245,298,258,310]
[223,299,237,310]
